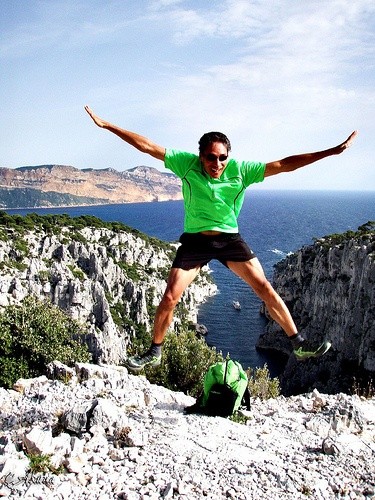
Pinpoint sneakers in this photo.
[292,339,332,361]
[127,350,162,371]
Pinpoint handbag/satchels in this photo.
[183,358,252,416]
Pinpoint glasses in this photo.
[202,153,229,161]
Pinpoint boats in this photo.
[233,301,241,310]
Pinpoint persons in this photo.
[84,103,359,375]
